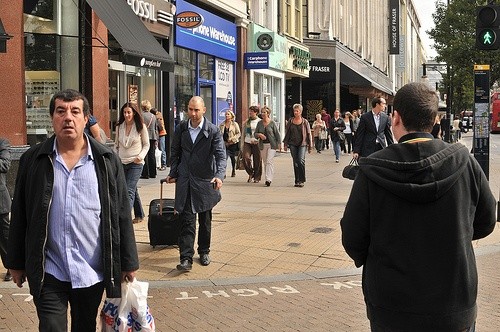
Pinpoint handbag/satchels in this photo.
[319,128,327,139]
[337,131,345,142]
[342,158,359,180]
[155,147,162,167]
[99,278,155,332]
[236,153,246,170]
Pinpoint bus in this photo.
[460,111,473,127]
[490,92,500,132]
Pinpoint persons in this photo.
[241,105,263,184]
[84,115,103,143]
[254,105,281,186]
[219,110,242,177]
[166,96,226,271]
[430,113,462,143]
[0,137,14,282]
[283,104,313,186]
[312,113,326,153]
[343,111,354,154]
[329,109,346,163]
[339,82,496,332]
[114,101,149,224]
[353,96,394,162]
[5,89,141,332]
[351,109,362,138]
[139,99,159,179]
[151,105,166,171]
[321,107,331,150]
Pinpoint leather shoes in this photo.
[200,254,210,266]
[4,272,13,281]
[177,259,191,270]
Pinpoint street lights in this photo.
[421,63,450,142]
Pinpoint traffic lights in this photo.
[476,6,500,50]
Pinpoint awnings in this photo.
[71,0,174,73]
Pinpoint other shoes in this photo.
[254,181,258,183]
[232,172,235,176]
[161,167,165,171]
[266,181,270,186]
[132,217,143,223]
[248,173,254,182]
[295,183,299,187]
[336,160,339,162]
[140,176,148,178]
[299,182,304,187]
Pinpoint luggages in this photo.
[147,179,180,248]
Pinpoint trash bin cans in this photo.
[4,146,31,199]
